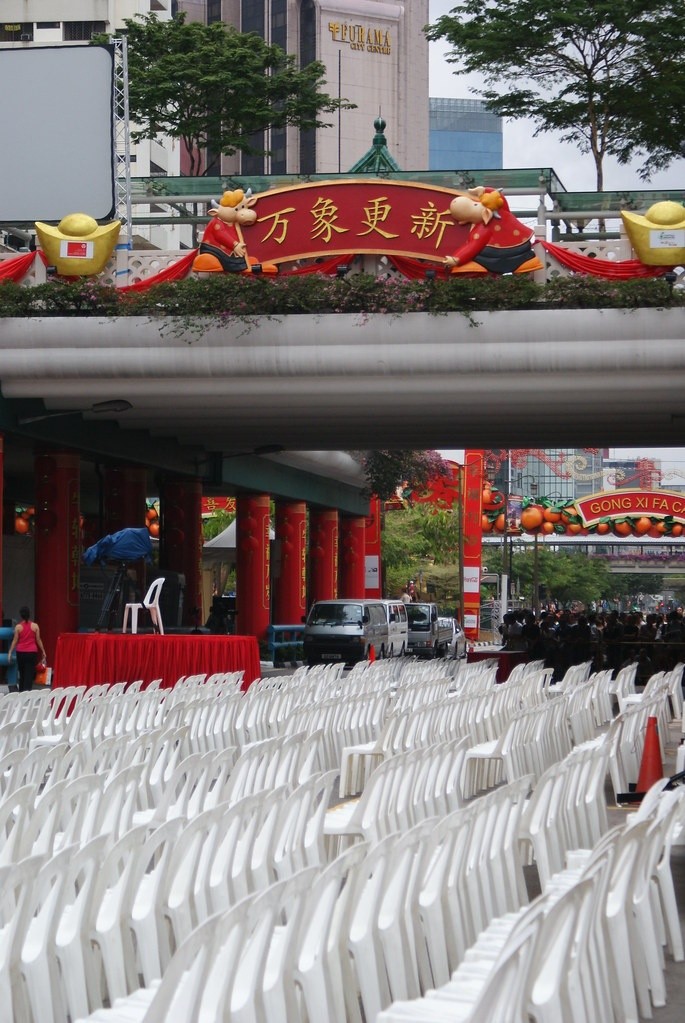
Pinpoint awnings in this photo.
[203,518,276,563]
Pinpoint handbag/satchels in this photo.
[34,660,52,685]
[35,657,46,672]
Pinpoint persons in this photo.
[498,597,685,684]
[205,596,228,634]
[8,606,46,692]
[399,588,411,603]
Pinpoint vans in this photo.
[380,600,408,658]
[302,599,388,665]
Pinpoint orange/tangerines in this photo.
[145,510,160,535]
[480,489,685,537]
[14,506,37,534]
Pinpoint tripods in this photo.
[94,560,160,635]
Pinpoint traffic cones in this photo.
[370,644,375,662]
[629,716,663,805]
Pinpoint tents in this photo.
[81,527,153,567]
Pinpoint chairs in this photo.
[122,577,165,635]
[0,655,685,1022]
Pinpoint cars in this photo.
[438,617,461,644]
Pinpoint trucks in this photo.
[404,603,453,656]
